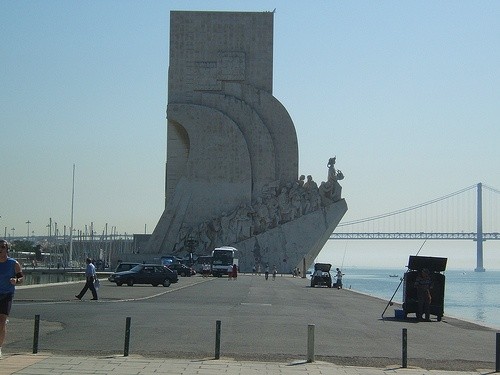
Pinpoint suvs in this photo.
[109,261,144,282]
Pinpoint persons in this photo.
[271,265,278,281]
[91,257,106,271]
[31,259,35,270]
[413,266,433,322]
[264,263,269,280]
[201,261,211,279]
[226,263,237,281]
[252,264,261,276]
[0,239,24,349]
[334,268,343,289]
[289,266,302,278]
[169,157,343,255]
[75,258,98,300]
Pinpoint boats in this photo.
[389,274,398,277]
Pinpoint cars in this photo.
[108,264,179,288]
[310,263,332,288]
[187,269,196,277]
[168,263,193,277]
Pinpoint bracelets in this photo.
[17,278,18,284]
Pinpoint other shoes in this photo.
[90,297,98,300]
[75,295,81,300]
[417,316,424,322]
[425,318,430,322]
[0,353,4,359]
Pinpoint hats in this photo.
[421,268,429,274]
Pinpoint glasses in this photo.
[0,245,6,248]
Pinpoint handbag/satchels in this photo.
[95,278,100,289]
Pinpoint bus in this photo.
[211,246,239,278]
